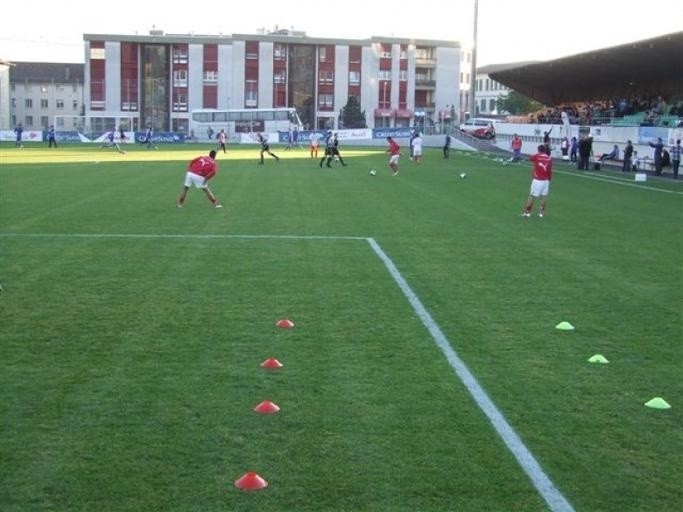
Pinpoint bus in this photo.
[185,107,303,141]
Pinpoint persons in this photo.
[46,125,57,148]
[13,123,24,148]
[206,126,452,177]
[510,96,682,179]
[137,127,154,151]
[99,127,127,155]
[174,149,222,209]
[518,145,552,218]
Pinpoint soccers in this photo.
[370,170,376,176]
[460,172,466,178]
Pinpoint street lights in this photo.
[381,81,388,127]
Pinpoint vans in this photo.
[458,118,506,139]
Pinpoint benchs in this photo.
[590,155,629,169]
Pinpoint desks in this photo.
[635,157,654,172]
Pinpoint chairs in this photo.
[508,99,682,125]
